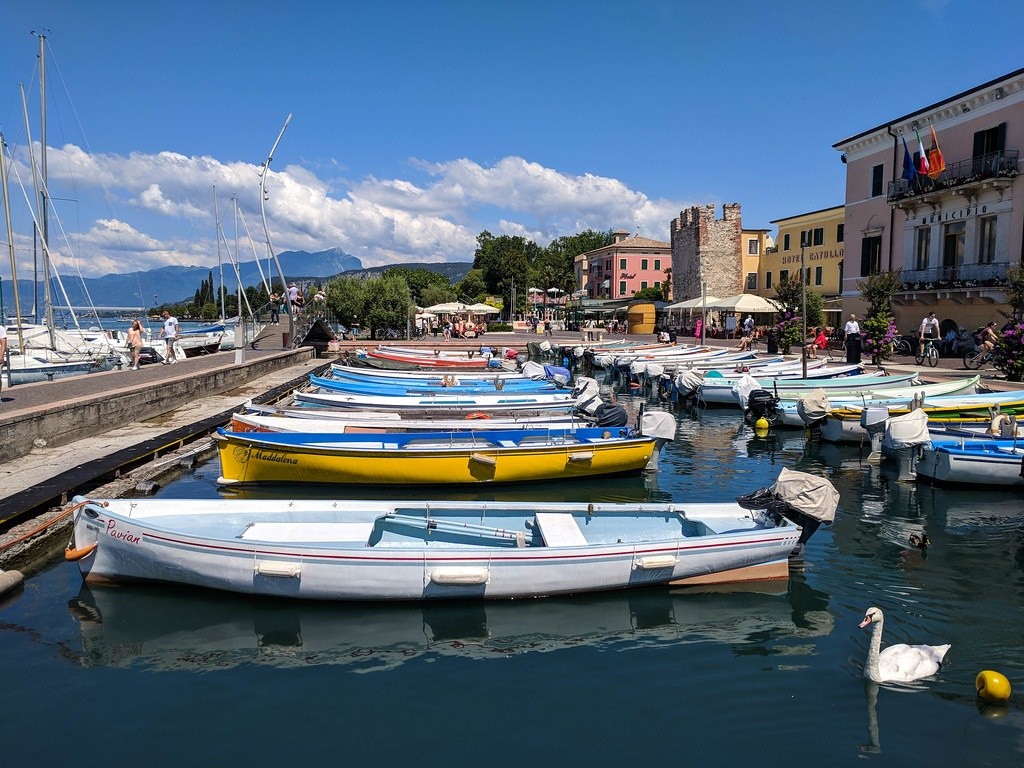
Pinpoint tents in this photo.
[421,302,499,323]
[664,294,798,339]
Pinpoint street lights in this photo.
[801,243,810,379]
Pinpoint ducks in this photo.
[909,533,931,548]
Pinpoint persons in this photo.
[525,314,553,336]
[918,312,942,356]
[0,325,8,403]
[974,321,998,363]
[270,283,326,325]
[744,314,754,336]
[350,315,360,342]
[124,319,143,371]
[586,318,628,341]
[843,313,862,340]
[157,310,178,365]
[806,328,826,359]
[735,326,760,351]
[422,316,501,341]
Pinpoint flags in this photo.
[928,124,945,180]
[916,129,930,175]
[901,137,917,185]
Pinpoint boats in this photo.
[530,341,865,408]
[216,348,657,494]
[773,372,1024,488]
[62,492,808,603]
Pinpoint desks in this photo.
[580,327,607,341]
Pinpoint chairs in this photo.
[746,329,758,352]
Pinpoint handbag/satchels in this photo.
[128,342,133,350]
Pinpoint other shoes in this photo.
[161,360,168,365]
[276,322,279,325]
[920,352,924,357]
[976,361,982,366]
[131,365,138,370]
[170,359,179,365]
[270,323,274,325]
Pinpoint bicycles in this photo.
[916,338,942,367]
[893,330,920,357]
[375,326,428,340]
[964,344,1001,370]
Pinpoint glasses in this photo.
[932,316,935,318]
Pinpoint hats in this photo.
[748,315,752,318]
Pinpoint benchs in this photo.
[805,337,834,360]
[998,447,1024,454]
[497,440,518,447]
[535,512,587,547]
[384,443,398,449]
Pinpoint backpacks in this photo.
[747,319,753,328]
[972,326,985,338]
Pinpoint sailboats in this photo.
[0,27,267,392]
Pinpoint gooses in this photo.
[856,607,951,682]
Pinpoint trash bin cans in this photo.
[846,333,862,364]
[669,328,677,342]
[767,329,778,354]
[283,333,289,348]
